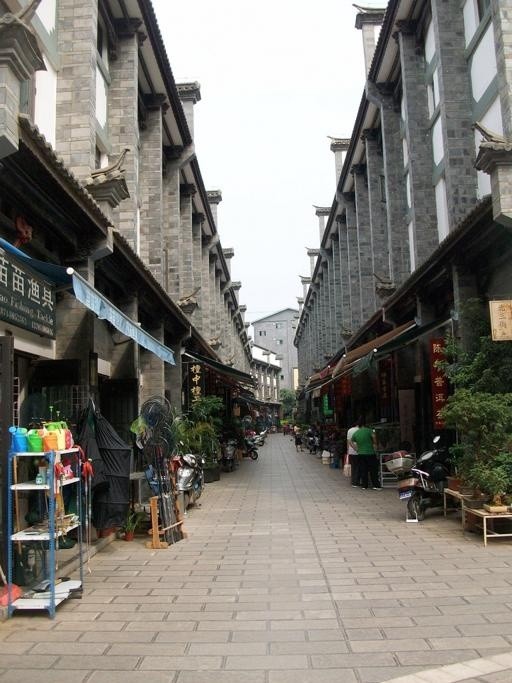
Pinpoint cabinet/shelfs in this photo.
[7,448,84,620]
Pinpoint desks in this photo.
[468,510,512,547]
[443,488,491,529]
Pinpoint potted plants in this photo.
[118,509,151,541]
[191,395,223,483]
[435,335,512,516]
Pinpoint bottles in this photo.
[27,547,50,578]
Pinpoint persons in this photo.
[346,418,363,487]
[350,419,382,490]
[283,420,318,454]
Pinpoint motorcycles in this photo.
[384,436,460,520]
[240,437,259,460]
[221,432,241,472]
[246,432,265,445]
[173,441,205,510]
